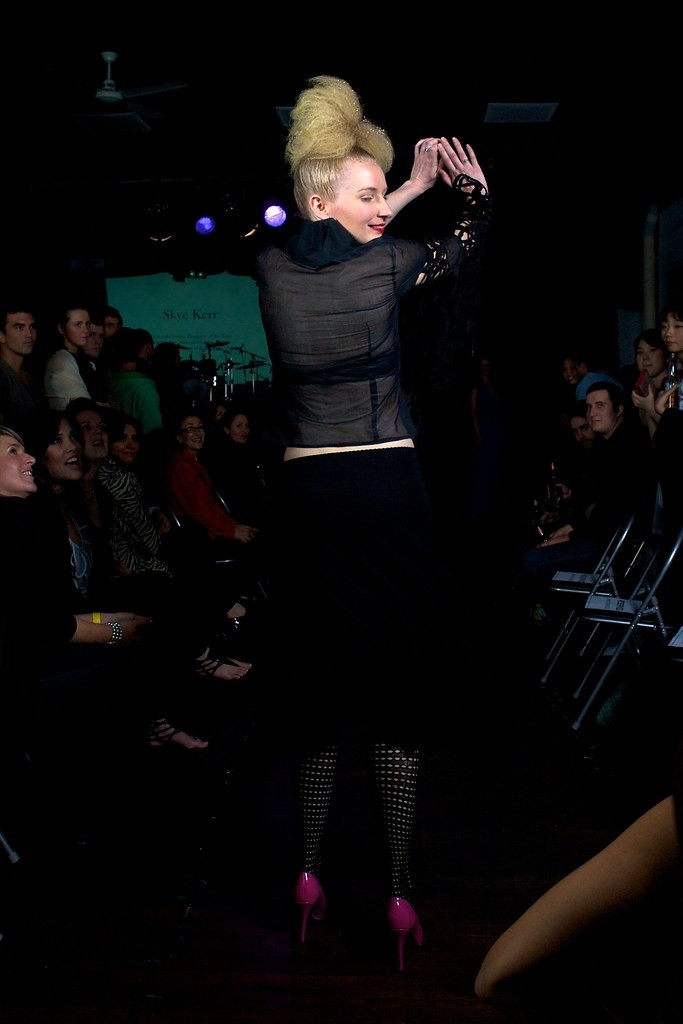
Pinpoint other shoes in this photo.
[193,647,251,680]
[147,721,208,750]
[532,608,554,624]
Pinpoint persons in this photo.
[505,304,682,665]
[0,307,257,759]
[475,789,682,1024]
[257,76,499,964]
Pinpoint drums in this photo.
[183,360,198,364]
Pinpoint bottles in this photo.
[548,461,561,502]
[530,497,540,533]
[661,353,679,410]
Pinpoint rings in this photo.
[633,400,635,402]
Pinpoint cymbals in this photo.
[208,340,230,347]
[222,362,241,365]
[171,342,192,350]
[242,361,264,369]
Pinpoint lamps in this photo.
[95,52,123,103]
[144,200,174,243]
[186,185,222,240]
[220,190,260,239]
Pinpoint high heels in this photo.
[296,871,326,943]
[390,897,423,969]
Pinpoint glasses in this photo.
[180,426,205,433]
[572,423,589,436]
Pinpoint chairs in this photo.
[538,512,683,731]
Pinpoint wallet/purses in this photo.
[634,369,659,401]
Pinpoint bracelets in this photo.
[105,622,124,648]
[92,612,101,625]
